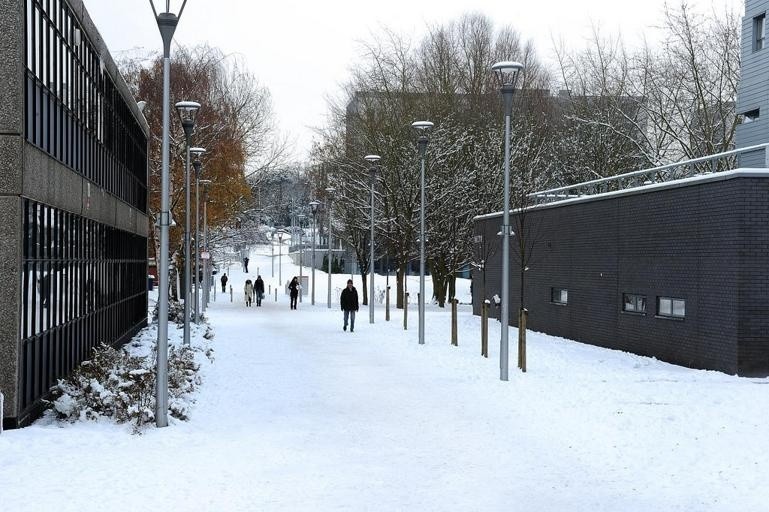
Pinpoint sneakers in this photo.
[343,325,354,332]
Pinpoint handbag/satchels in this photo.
[296,283,303,291]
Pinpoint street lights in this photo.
[186,147,209,326]
[197,176,218,318]
[486,59,529,379]
[266,186,338,308]
[408,117,436,343]
[361,153,380,324]
[138,2,193,428]
[172,99,205,351]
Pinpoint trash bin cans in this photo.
[148,275,155,290]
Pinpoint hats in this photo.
[347,279,353,284]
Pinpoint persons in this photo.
[244,276,264,307]
[341,280,359,332]
[288,277,299,310]
[221,273,228,293]
